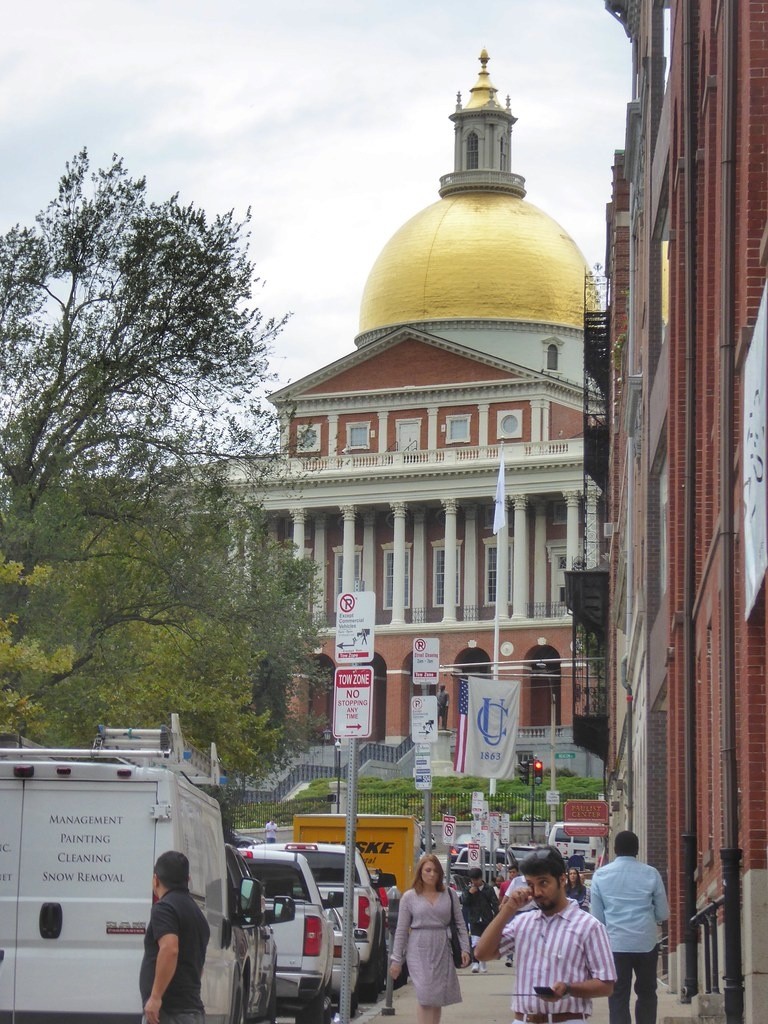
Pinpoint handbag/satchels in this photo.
[448,884,474,968]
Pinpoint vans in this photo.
[548,822,606,871]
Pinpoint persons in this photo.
[460,862,592,975]
[589,831,670,1024]
[138,851,210,1024]
[474,845,618,1024]
[264,818,278,844]
[389,854,471,1024]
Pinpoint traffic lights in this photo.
[518,760,530,787]
[326,795,336,803]
[535,760,543,785]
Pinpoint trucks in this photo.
[0,713,265,1024]
[292,813,422,897]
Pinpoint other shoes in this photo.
[471,963,479,972]
[480,961,488,973]
[505,959,513,967]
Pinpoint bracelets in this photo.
[561,981,572,1000]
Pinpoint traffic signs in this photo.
[410,695,439,743]
[335,591,376,663]
[332,666,373,740]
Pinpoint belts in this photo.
[515,1012,588,1024]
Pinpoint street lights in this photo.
[335,742,341,814]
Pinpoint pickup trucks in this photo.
[247,842,398,1007]
[451,847,518,885]
[237,848,335,1023]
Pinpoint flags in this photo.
[463,675,520,782]
[453,677,469,775]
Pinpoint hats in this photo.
[156,850,190,892]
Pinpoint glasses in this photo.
[569,872,578,876]
[523,849,559,865]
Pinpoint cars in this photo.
[448,832,474,863]
[256,846,296,1023]
[323,899,361,1016]
[508,843,535,864]
